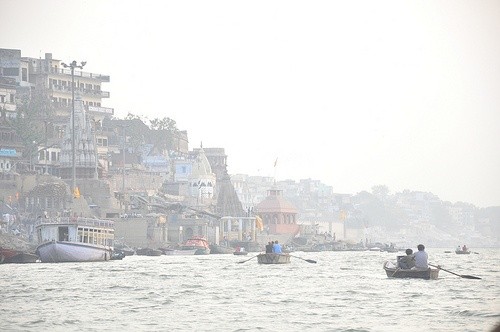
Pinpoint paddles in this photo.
[427,263,481,280]
[288,254,317,264]
[234,254,257,263]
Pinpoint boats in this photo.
[233,245,248,255]
[111,244,176,260]
[383,256,482,281]
[0,247,39,263]
[444,248,480,255]
[278,241,406,254]
[234,253,318,265]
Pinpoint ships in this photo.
[32,206,115,262]
[176,237,211,255]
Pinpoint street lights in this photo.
[60,58,88,203]
[115,122,133,207]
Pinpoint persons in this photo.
[270,240,275,246]
[235,244,241,253]
[272,241,282,254]
[462,244,468,252]
[455,245,461,252]
[265,241,272,254]
[35,208,98,227]
[410,244,428,271]
[397,249,416,270]
